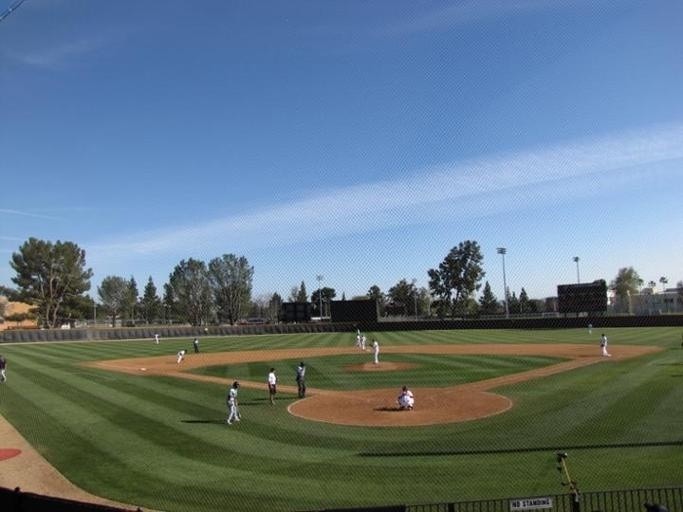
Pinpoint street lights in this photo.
[573,256,581,283]
[316,273,324,321]
[659,275,668,315]
[495,247,510,319]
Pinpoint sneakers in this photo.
[226,396,306,425]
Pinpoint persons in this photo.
[191,337,200,354]
[599,332,609,358]
[176,349,187,364]
[267,367,278,405]
[368,339,379,363]
[153,332,160,346]
[354,327,365,351]
[224,381,241,424]
[0,354,6,383]
[396,386,413,411]
[294,361,306,399]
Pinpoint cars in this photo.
[59,317,267,329]
